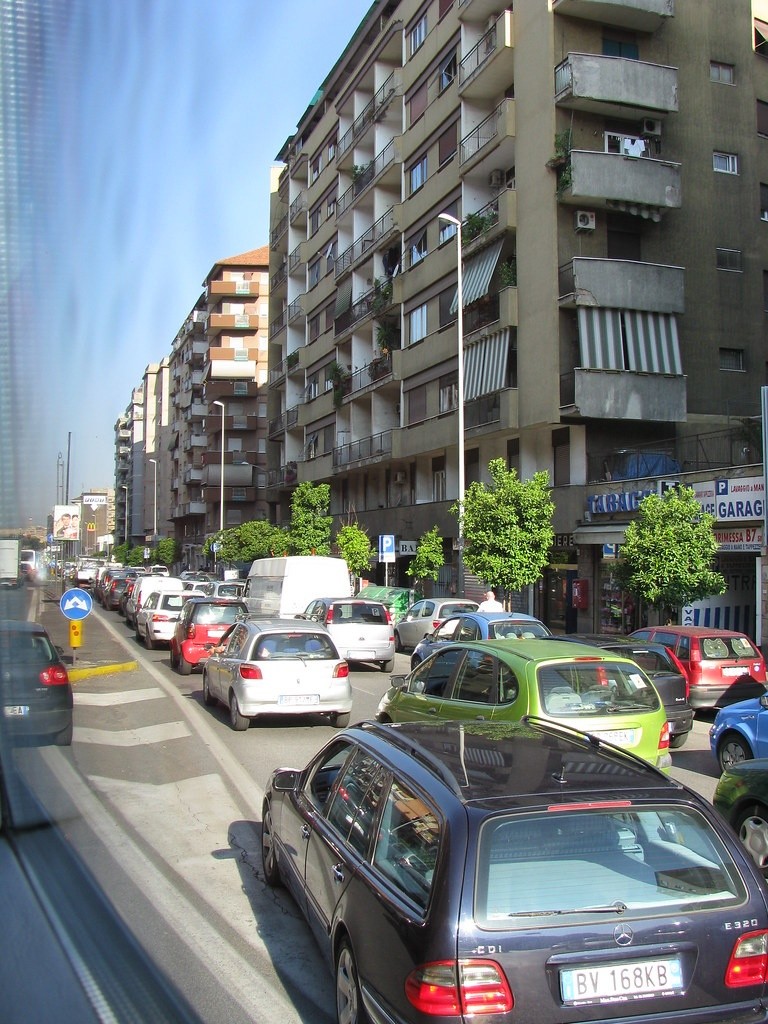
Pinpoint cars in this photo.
[202,620,352,731]
[21,563,35,582]
[375,611,768,890]
[0,620,75,751]
[49,554,479,676]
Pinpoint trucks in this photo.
[236,556,353,618]
[0,538,24,590]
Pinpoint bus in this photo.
[21,550,39,569]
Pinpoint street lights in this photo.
[80,514,97,554]
[214,400,225,576]
[122,485,128,541]
[437,214,466,596]
[149,458,158,545]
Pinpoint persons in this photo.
[199,564,210,571]
[58,514,78,537]
[208,645,226,654]
[181,563,188,571]
[476,591,503,612]
[73,565,78,588]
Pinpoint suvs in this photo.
[260,715,767,1023]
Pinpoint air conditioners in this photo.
[489,169,502,186]
[573,209,595,230]
[394,471,406,484]
[641,116,664,137]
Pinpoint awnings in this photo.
[464,327,510,402]
[578,305,683,374]
[200,362,211,384]
[168,431,179,449]
[449,235,506,314]
